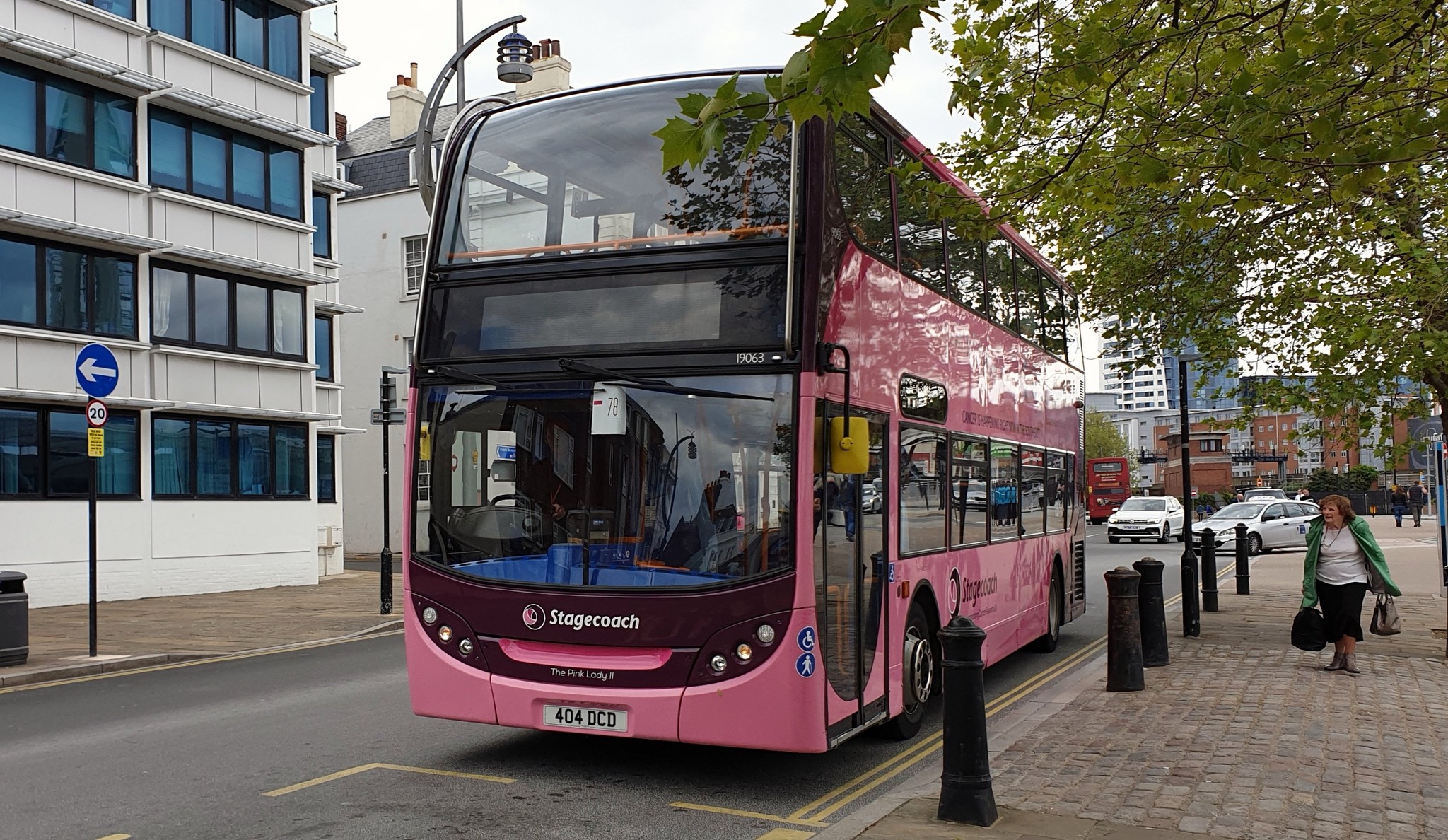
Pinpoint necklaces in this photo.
[1321,520,1345,549]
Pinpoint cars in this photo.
[1085,510,1090,521]
[861,482,883,513]
[950,481,987,511]
[1243,486,1290,502]
[1192,496,1323,557]
[1106,495,1186,544]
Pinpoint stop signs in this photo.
[1256,478,1263,487]
[1443,442,1448,461]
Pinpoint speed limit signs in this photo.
[85,399,110,428]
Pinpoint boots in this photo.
[1396,519,1402,528]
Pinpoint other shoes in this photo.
[1414,523,1421,527]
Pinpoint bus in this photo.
[1087,457,1132,526]
[398,67,1091,761]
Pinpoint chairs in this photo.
[523,223,922,277]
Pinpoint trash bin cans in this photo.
[0,571,29,667]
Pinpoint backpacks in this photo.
[1209,506,1214,513]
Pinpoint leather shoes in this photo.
[1324,651,1345,671]
[1342,653,1360,673]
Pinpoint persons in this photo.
[1301,495,1402,673]
[1390,486,1406,527]
[1406,481,1428,527]
[1196,504,1205,522]
[1233,493,1243,503]
[838,474,858,541]
[520,411,587,533]
[1206,503,1214,518]
[1294,489,1317,504]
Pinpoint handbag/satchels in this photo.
[1368,593,1401,636]
[1291,606,1327,651]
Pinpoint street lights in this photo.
[1177,352,1213,637]
[414,0,534,253]
